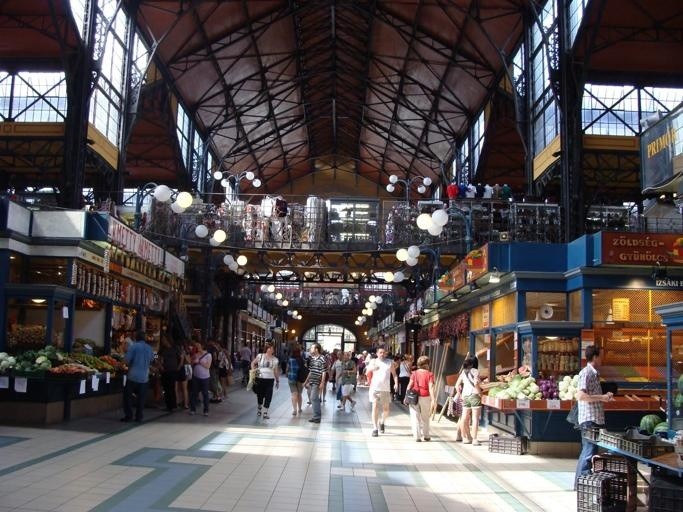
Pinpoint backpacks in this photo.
[217,351,230,378]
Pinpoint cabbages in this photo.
[0,345,55,371]
[488,374,542,401]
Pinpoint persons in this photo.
[452,356,484,445]
[239,342,437,442]
[571,345,616,492]
[118,332,231,424]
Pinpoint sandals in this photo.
[264,413,270,419]
[257,407,261,416]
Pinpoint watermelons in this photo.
[674,374,683,408]
[639,415,668,435]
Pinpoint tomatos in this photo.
[101,355,128,372]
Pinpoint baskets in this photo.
[577,424,683,511]
[488,433,528,455]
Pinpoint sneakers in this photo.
[372,430,378,437]
[379,422,384,434]
[350,401,356,410]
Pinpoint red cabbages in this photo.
[536,378,560,400]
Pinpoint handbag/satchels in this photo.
[296,366,310,383]
[566,401,578,425]
[405,389,418,405]
[184,364,193,381]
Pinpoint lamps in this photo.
[154,168,304,326]
[352,175,450,327]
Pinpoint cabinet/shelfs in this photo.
[510,205,559,242]
[586,207,631,236]
[453,200,513,242]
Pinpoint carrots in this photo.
[48,367,92,373]
